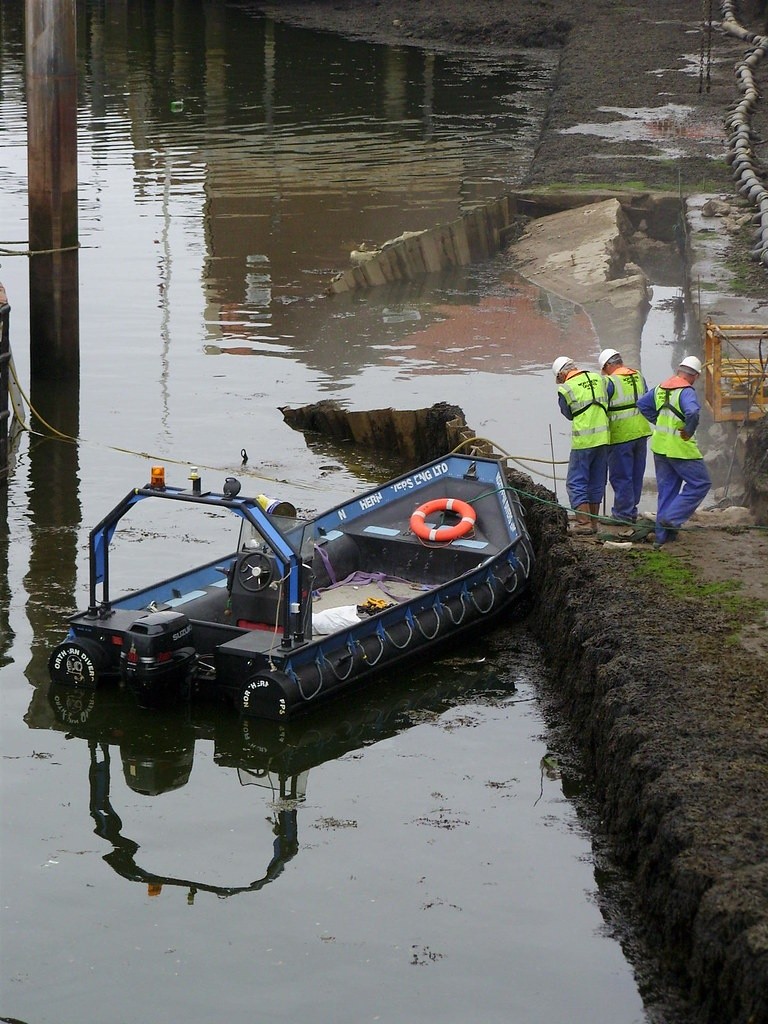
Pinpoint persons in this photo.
[636,355,713,549]
[598,348,652,527]
[552,356,611,536]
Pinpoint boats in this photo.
[49,622,512,907]
[49,452,538,721]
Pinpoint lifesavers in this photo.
[410,498,476,542]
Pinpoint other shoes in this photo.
[653,542,663,548]
[601,515,632,526]
[659,519,679,534]
[633,518,636,524]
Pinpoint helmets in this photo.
[552,356,573,384]
[679,356,702,374]
[598,348,620,375]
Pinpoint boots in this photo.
[569,503,593,535]
[590,503,598,532]
